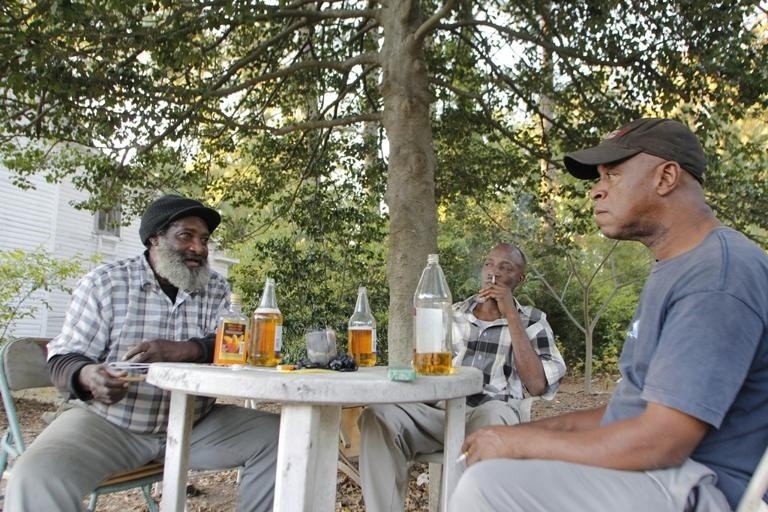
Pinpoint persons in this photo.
[357,241,567,511]
[0,193,282,511]
[442,118,768,511]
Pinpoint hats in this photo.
[564,118,707,185]
[139,194,221,248]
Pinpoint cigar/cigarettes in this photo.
[117,376,145,382]
[457,450,468,464]
[491,274,496,285]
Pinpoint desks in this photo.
[148,362,485,512]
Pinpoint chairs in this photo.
[0,338,165,511]
[395,365,562,510]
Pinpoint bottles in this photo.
[250,277,283,366]
[412,253,453,374]
[347,286,377,366]
[213,293,250,365]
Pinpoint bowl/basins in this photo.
[108,361,149,381]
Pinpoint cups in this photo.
[305,327,336,364]
